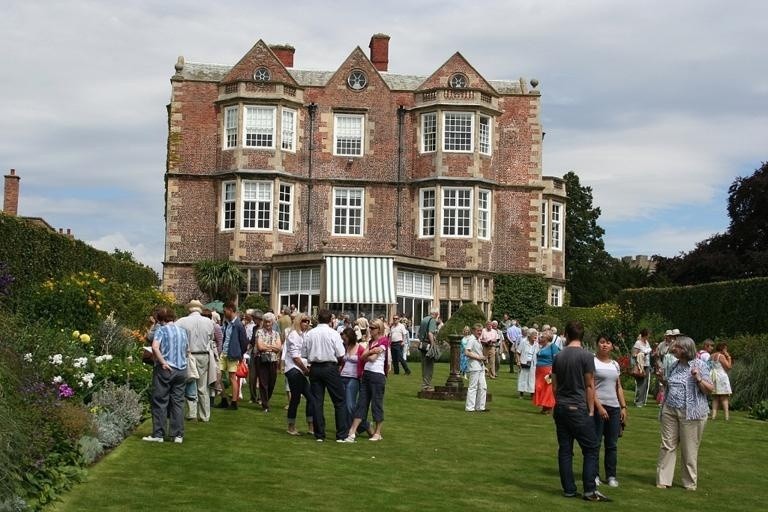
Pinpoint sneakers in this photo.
[142,436,163,443]
[174,436,183,443]
[565,476,619,503]
[287,429,383,443]
[227,405,238,410]
[216,403,228,408]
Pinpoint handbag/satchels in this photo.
[236,361,248,378]
[418,340,431,351]
[186,356,200,383]
[521,360,531,369]
[256,353,272,367]
[627,359,646,378]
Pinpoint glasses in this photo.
[369,326,378,330]
[302,319,310,324]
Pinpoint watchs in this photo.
[620,405,627,409]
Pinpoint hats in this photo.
[665,329,684,336]
[185,300,203,308]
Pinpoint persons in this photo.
[631,328,733,421]
[583,332,628,486]
[657,337,715,489]
[552,320,613,502]
[418,309,438,390]
[461,317,571,416]
[141,299,413,442]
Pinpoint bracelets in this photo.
[697,379,702,385]
